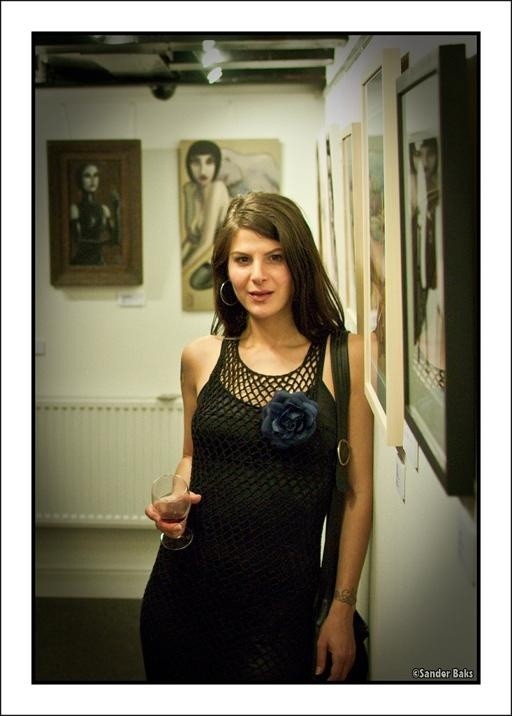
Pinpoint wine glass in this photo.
[151,471,195,552]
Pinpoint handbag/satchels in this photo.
[313,579,369,684]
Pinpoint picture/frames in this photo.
[336,41,478,497]
[47,137,144,288]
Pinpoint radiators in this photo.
[35,396,183,530]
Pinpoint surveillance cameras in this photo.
[152,82,175,100]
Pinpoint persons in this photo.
[181,138,234,295]
[65,158,126,264]
[136,191,384,686]
